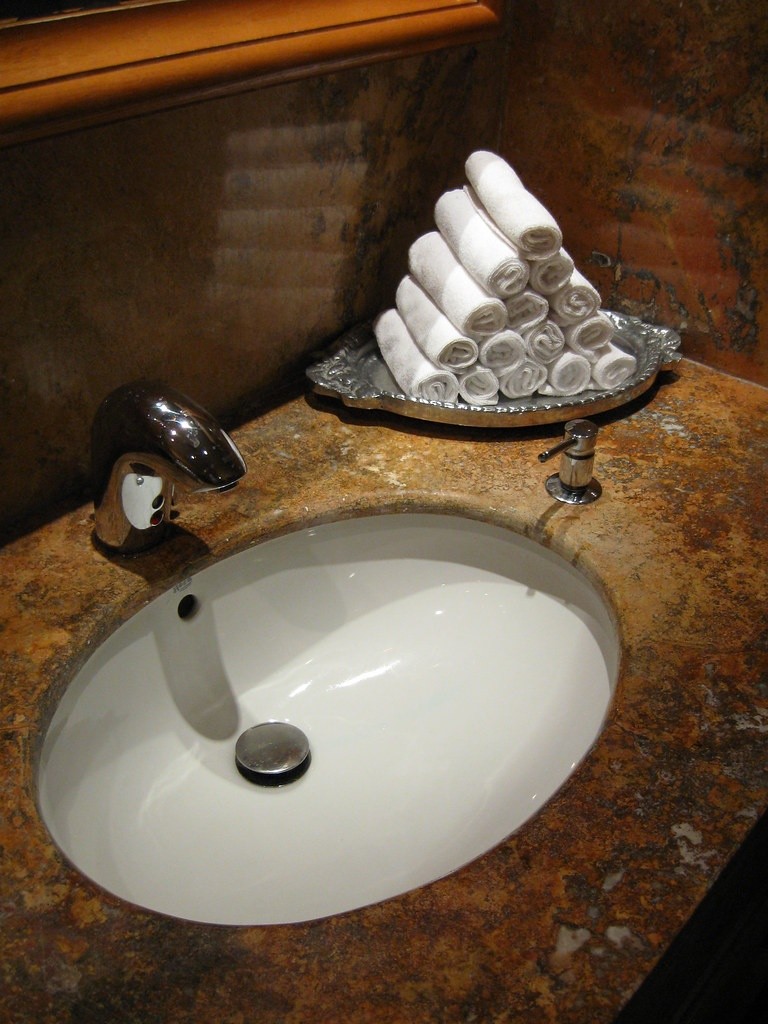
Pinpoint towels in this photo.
[373,150,638,406]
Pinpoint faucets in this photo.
[88,377,247,558]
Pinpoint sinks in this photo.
[29,499,624,928]
